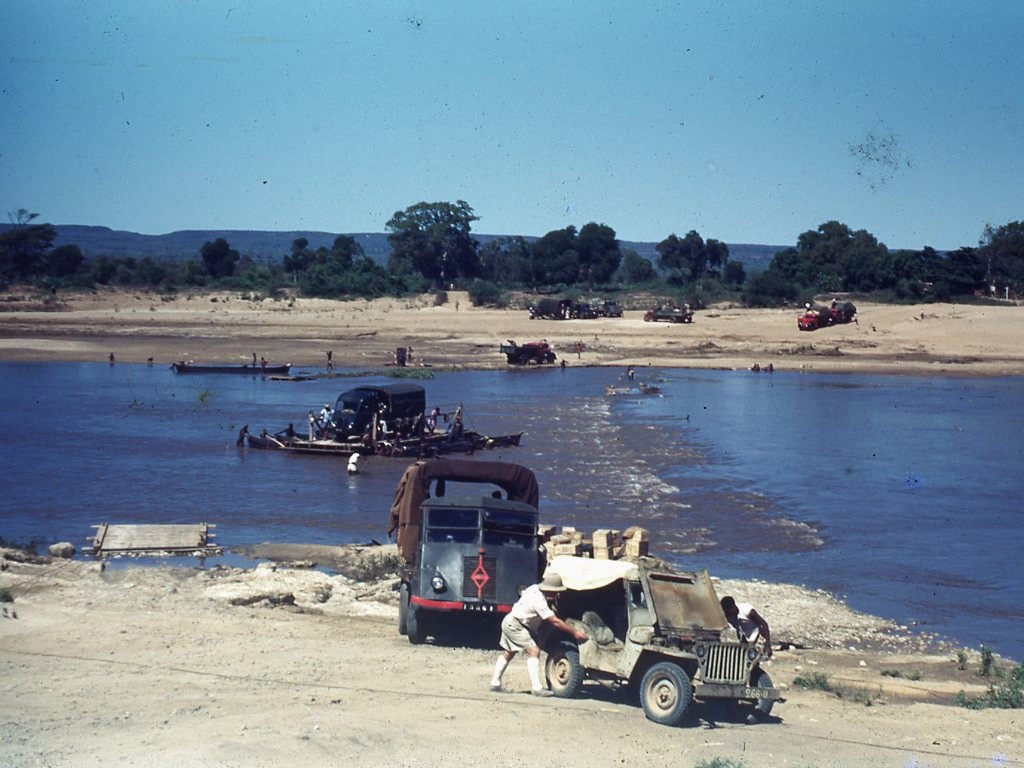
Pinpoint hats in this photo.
[539,575,567,592]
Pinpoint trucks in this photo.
[797,300,857,330]
[527,298,623,319]
[501,338,557,364]
[384,455,546,645]
[645,302,693,323]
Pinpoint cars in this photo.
[540,554,787,726]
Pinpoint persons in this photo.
[576,343,582,359]
[561,358,569,368]
[252,353,256,364]
[327,351,333,370]
[348,453,363,474]
[311,403,442,440]
[507,339,517,347]
[455,300,460,310]
[831,299,838,308]
[109,352,114,361]
[260,429,268,438]
[274,423,299,437]
[751,362,773,371]
[721,597,773,657]
[261,357,268,366]
[491,490,502,498]
[490,572,588,697]
[237,423,249,447]
[684,304,692,313]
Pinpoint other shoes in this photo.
[531,687,553,696]
[490,684,513,693]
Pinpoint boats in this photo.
[173,362,293,375]
[247,382,527,458]
